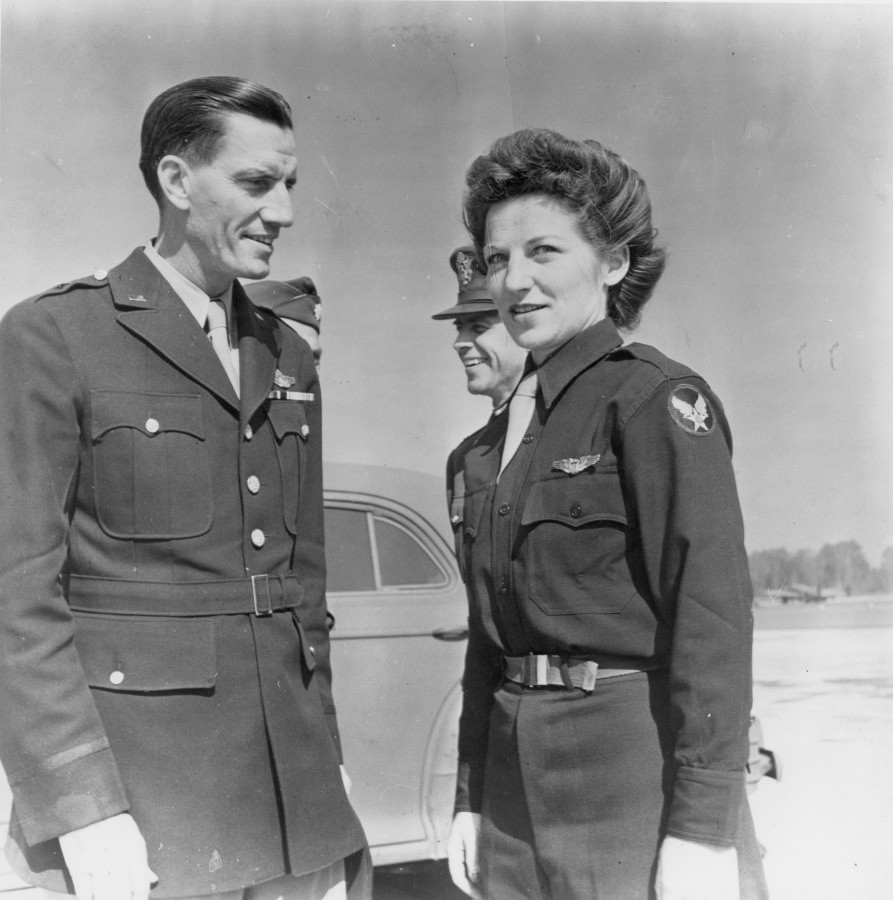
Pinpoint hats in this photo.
[431,246,498,320]
[242,276,322,334]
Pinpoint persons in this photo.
[431,245,532,583]
[448,130,770,900]
[0,77,370,900]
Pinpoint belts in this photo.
[503,653,670,692]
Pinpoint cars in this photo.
[323,461,778,868]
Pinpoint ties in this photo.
[496,370,539,484]
[207,301,241,401]
